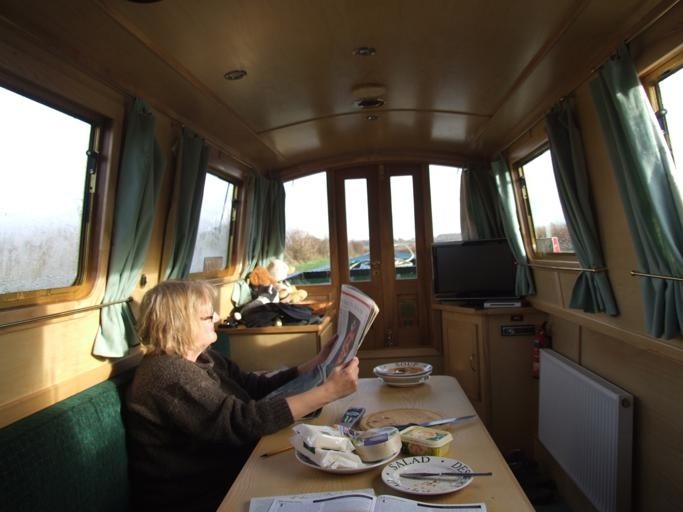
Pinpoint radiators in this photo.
[536,347,635,511]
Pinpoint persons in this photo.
[122,275,361,512]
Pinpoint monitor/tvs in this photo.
[430,237,517,307]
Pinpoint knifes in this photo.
[399,471,493,479]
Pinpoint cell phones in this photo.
[341,407,365,428]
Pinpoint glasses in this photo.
[200,311,215,322]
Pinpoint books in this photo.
[267,494,487,512]
[248,487,376,512]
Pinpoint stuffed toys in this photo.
[233,257,308,320]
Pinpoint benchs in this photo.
[1,372,129,510]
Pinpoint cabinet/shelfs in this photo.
[216,309,333,374]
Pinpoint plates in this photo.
[296,431,403,474]
[373,361,433,387]
[381,454,474,497]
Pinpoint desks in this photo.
[430,296,546,459]
